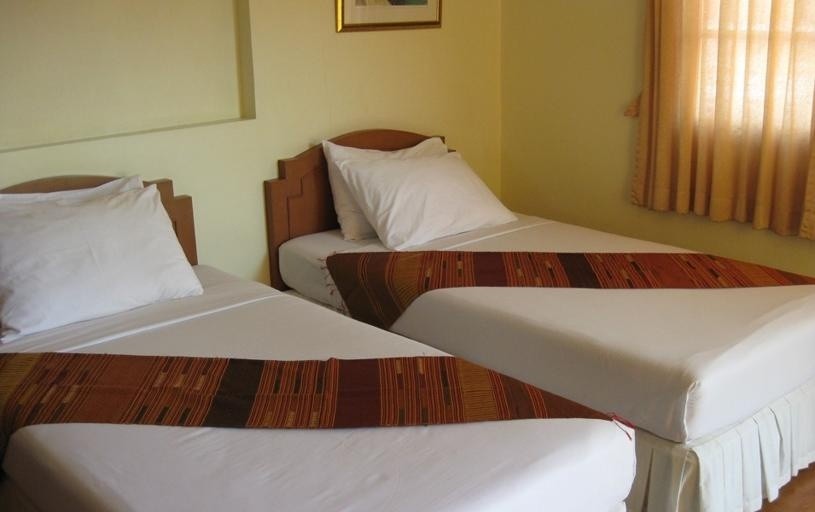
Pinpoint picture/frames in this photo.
[336,1,444,31]
